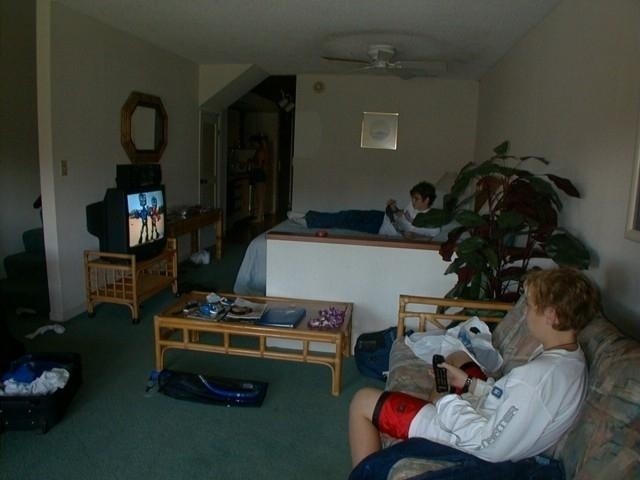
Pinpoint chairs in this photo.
[3,225,51,326]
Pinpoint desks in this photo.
[162,203,224,296]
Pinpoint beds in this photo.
[232,166,537,303]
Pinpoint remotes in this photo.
[433,355,448,393]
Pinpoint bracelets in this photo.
[463,375,472,392]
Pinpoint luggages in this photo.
[1,352,82,432]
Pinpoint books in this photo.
[188,298,306,329]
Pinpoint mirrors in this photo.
[118,90,170,167]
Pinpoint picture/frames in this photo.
[360,110,400,153]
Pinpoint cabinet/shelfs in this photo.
[82,236,183,325]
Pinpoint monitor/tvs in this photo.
[86,185,167,264]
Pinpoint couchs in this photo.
[374,274,640,480]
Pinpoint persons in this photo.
[286,180,436,235]
[348,268,603,469]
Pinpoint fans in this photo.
[318,40,451,81]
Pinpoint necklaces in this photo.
[546,342,576,350]
